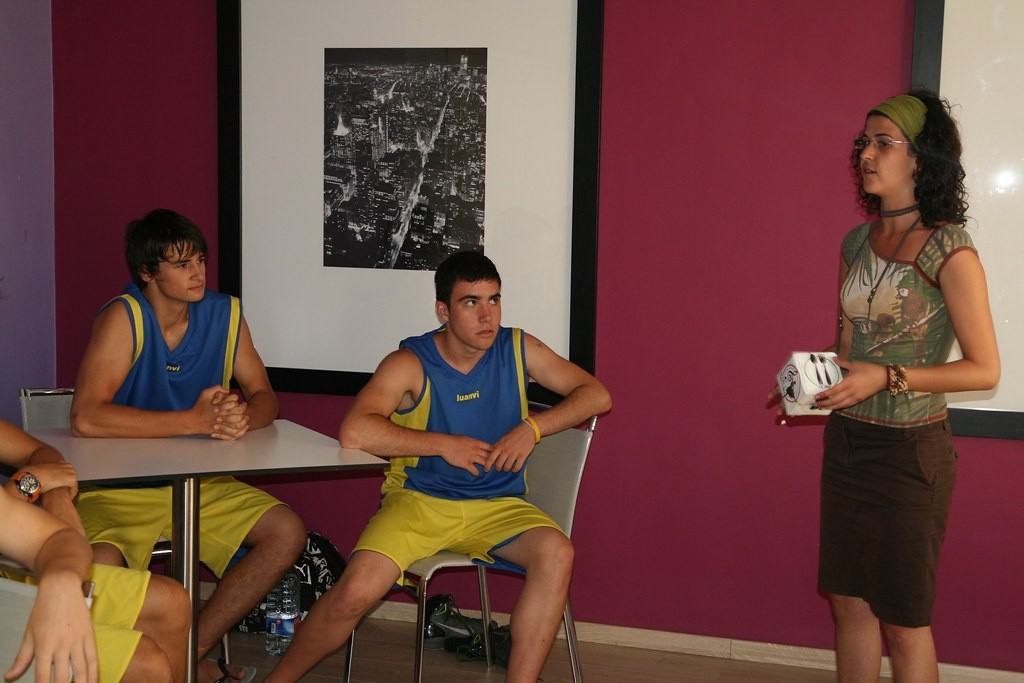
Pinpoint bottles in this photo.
[265,574,301,656]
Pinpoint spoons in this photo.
[810,354,823,385]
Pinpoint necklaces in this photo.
[879,203,918,218]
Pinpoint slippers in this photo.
[203,658,256,683]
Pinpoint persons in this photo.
[69,207,306,683]
[764,86,1002,683]
[0,417,192,682]
[261,251,612,683]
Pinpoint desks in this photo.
[10,417,392,682]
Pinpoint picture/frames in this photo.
[210,0,606,408]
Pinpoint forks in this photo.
[818,354,832,385]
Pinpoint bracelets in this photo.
[886,363,909,397]
[523,416,541,444]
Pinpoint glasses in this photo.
[856,138,912,151]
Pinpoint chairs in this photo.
[14,388,230,668]
[342,398,598,682]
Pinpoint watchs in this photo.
[9,470,42,505]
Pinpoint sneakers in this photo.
[457,624,512,669]
[423,593,497,650]
